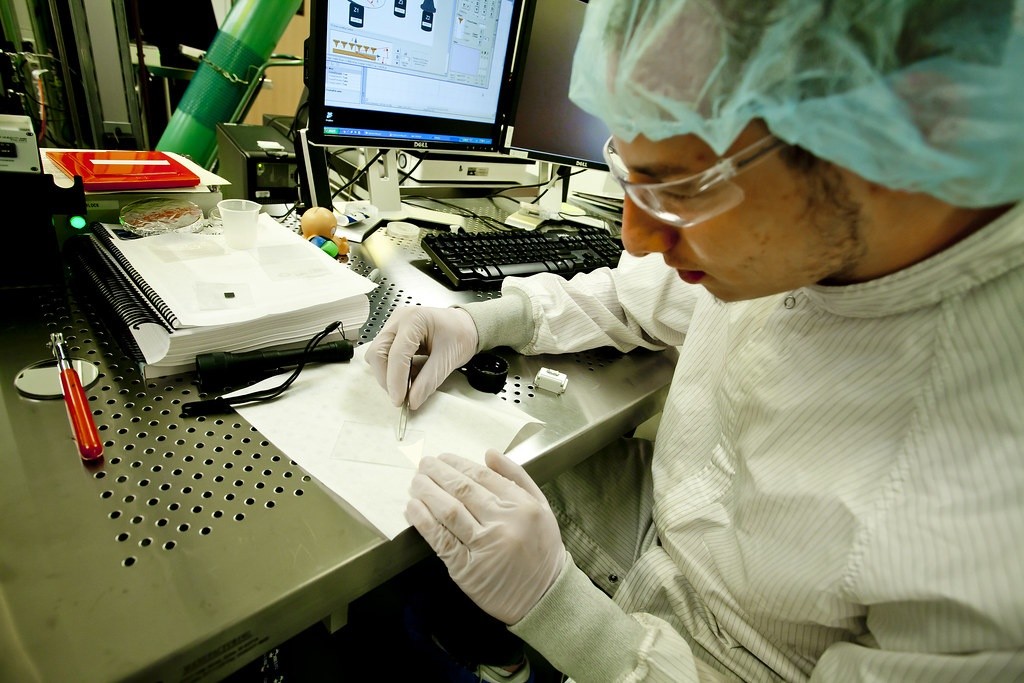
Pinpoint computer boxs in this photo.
[216,122,300,203]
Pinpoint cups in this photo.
[217,198,262,250]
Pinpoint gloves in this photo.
[402,446,566,626]
[364,304,479,413]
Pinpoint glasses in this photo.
[597,128,784,231]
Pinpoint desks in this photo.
[0,198,688,682]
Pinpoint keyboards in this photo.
[421,225,622,287]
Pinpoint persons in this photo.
[359,0,1024,682]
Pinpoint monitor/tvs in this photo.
[304,0,537,153]
[499,0,612,171]
